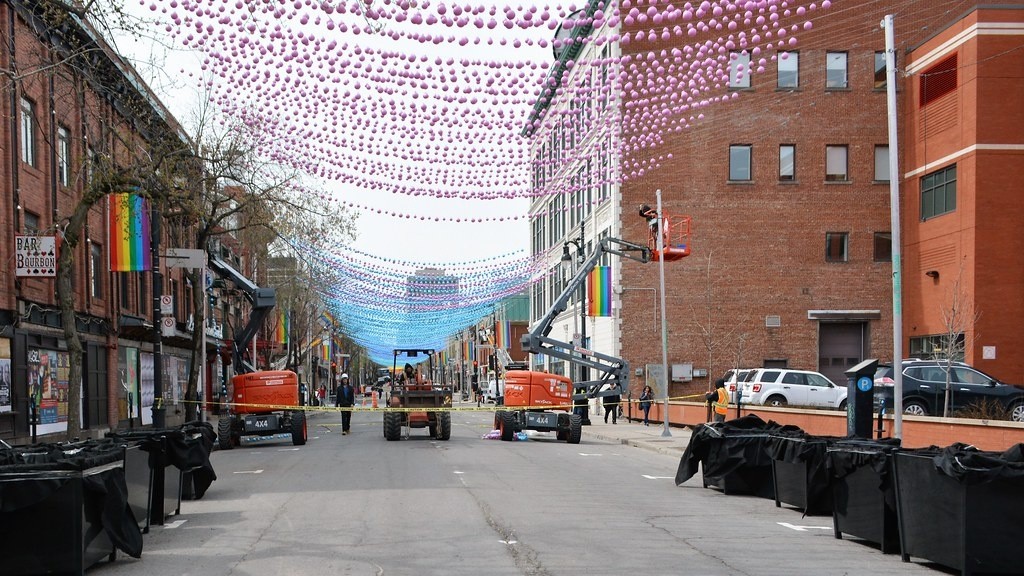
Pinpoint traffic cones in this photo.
[362,398,366,406]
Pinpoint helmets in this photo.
[341,373,348,379]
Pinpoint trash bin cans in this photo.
[698,414,1024,576]
[0,421,216,576]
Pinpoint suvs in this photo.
[871,356,1024,422]
[738,368,849,411]
[721,370,749,404]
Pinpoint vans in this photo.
[487,381,504,402]
[365,387,373,398]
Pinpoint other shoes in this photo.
[613,422,617,424]
[644,423,649,426]
[605,418,607,423]
[342,430,351,434]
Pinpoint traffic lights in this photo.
[312,359,318,373]
[331,363,336,375]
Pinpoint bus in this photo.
[376,368,390,383]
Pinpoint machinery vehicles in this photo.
[494,209,693,446]
[383,348,452,442]
[208,244,308,448]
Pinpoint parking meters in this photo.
[735,389,742,419]
[627,390,631,424]
[31,394,37,443]
[876,398,885,439]
[129,392,135,428]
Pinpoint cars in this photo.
[301,382,320,405]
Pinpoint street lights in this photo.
[561,240,591,425]
[479,328,500,405]
[203,146,213,425]
[455,332,464,399]
[150,182,190,431]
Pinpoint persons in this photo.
[706,378,729,423]
[574,387,587,415]
[399,363,415,385]
[639,204,658,240]
[0,364,10,389]
[317,382,326,407]
[603,383,620,424]
[640,386,653,426]
[477,387,483,396]
[185,389,199,408]
[378,389,383,399]
[334,373,354,435]
